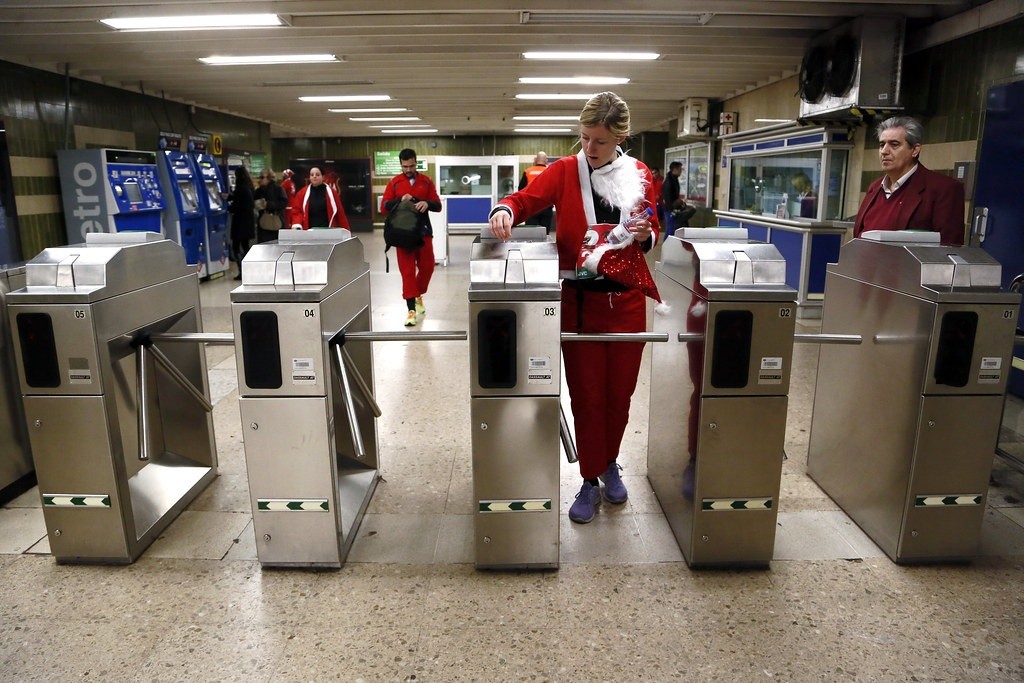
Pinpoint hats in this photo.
[283,169,294,179]
[586,236,671,316]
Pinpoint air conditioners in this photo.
[676,97,708,140]
[781,19,903,122]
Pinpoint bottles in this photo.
[606,207,653,245]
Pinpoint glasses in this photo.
[260,176,270,179]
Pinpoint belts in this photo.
[563,277,628,293]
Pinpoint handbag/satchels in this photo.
[260,213,282,231]
[674,206,695,221]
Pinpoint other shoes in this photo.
[234,274,242,280]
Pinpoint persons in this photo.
[792,173,817,218]
[381,148,442,325]
[518,151,553,236]
[223,165,351,280]
[853,117,965,246]
[661,161,685,240]
[651,169,665,218]
[488,92,660,521]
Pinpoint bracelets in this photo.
[490,206,511,217]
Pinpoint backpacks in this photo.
[384,197,424,249]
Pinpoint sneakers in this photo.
[598,461,628,504]
[415,296,425,313]
[682,467,695,499]
[405,310,416,325]
[569,481,601,522]
